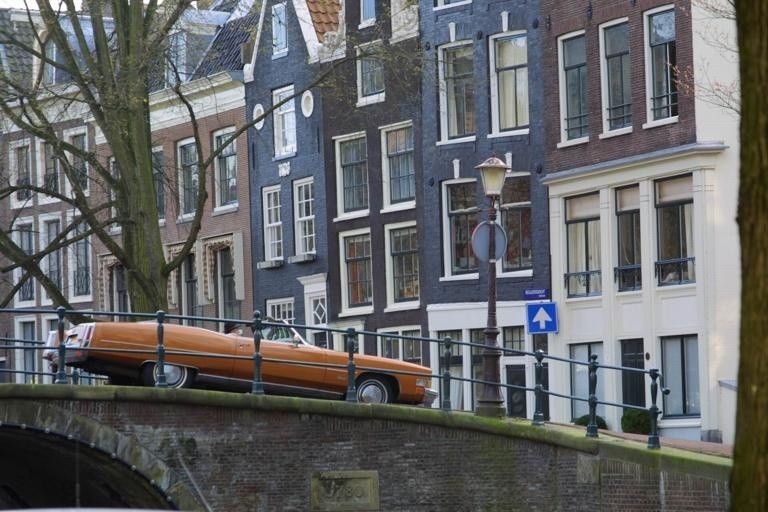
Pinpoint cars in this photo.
[46,315,434,410]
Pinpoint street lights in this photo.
[471,155,515,416]
